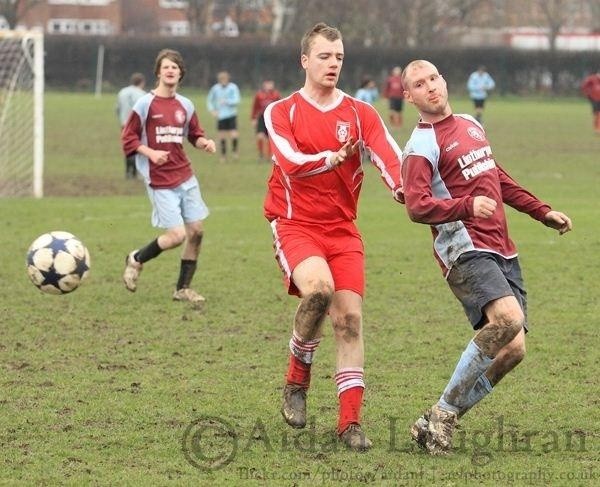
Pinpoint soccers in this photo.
[27,231,89,295]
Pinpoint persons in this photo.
[581,71,599,131]
[350,73,379,105]
[206,68,243,163]
[396,58,572,457]
[120,47,220,309]
[383,63,408,132]
[114,73,148,188]
[258,21,408,453]
[250,77,282,163]
[465,63,496,120]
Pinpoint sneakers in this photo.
[172,286,204,302]
[282,383,308,427]
[123,250,142,292]
[410,404,457,457]
[339,424,373,450]
